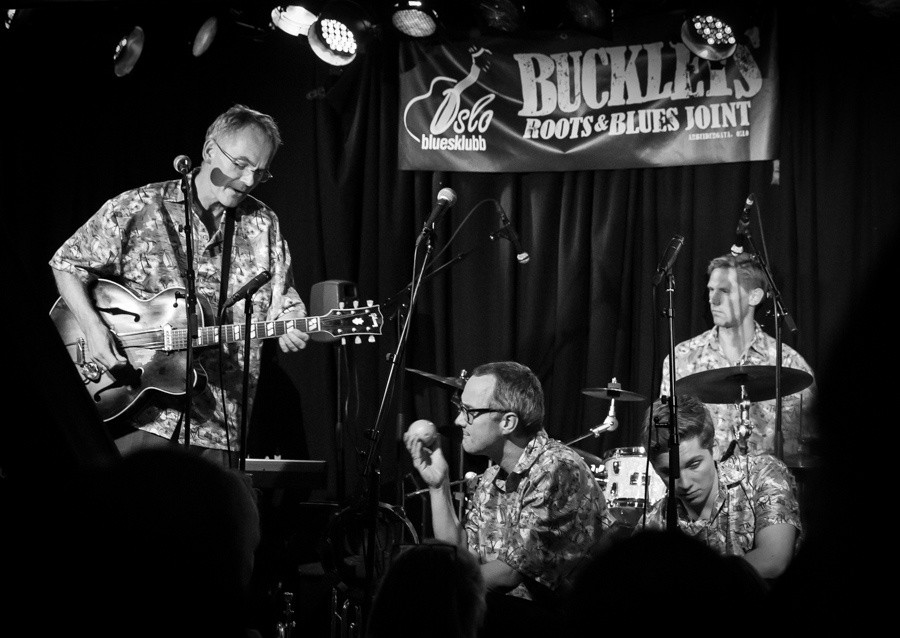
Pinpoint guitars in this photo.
[48,278,384,423]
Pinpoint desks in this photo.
[238,458,330,478]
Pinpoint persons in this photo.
[656,249,816,470]
[630,393,804,580]
[403,362,618,619]
[45,103,311,462]
[0,442,900,637]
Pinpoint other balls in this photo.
[408,420,438,446]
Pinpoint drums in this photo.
[602,447,668,528]
[321,501,420,597]
[569,446,608,488]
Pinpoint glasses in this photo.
[213,138,273,183]
[460,403,513,422]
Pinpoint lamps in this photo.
[190,8,243,56]
[389,1,447,39]
[266,0,385,68]
[678,1,763,63]
[63,5,145,78]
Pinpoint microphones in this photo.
[731,195,754,256]
[653,234,684,286]
[224,272,272,308]
[496,201,529,263]
[416,188,458,246]
[174,154,191,173]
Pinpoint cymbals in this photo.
[784,452,823,470]
[582,388,647,402]
[674,365,814,404]
[404,367,466,391]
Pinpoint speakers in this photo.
[311,281,357,341]
